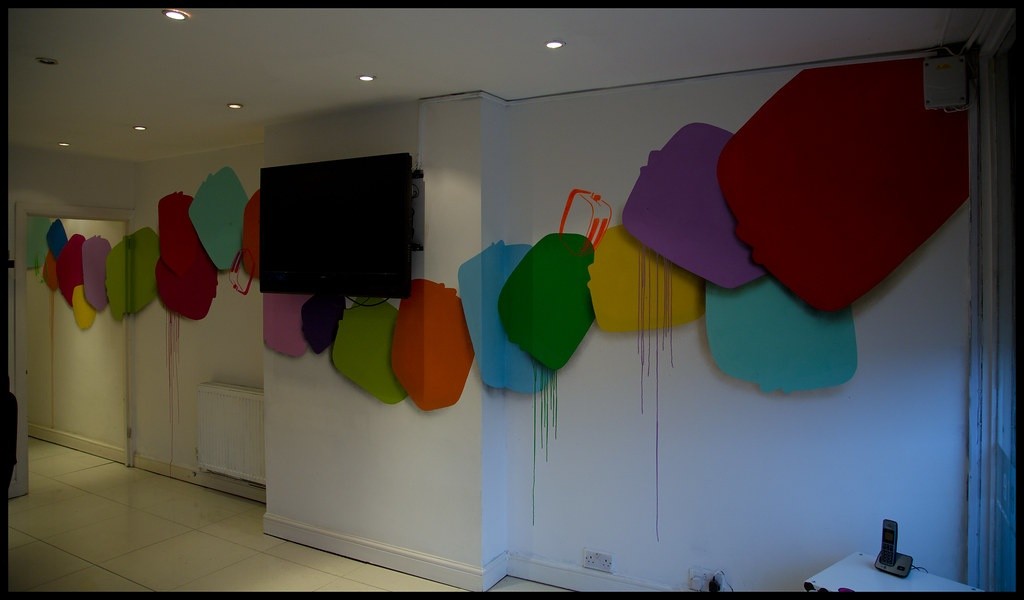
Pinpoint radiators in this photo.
[198,383,266,486]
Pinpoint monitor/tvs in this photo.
[259,152,414,298]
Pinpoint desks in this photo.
[802,552,985,592]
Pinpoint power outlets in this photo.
[689,568,726,592]
[583,548,614,573]
